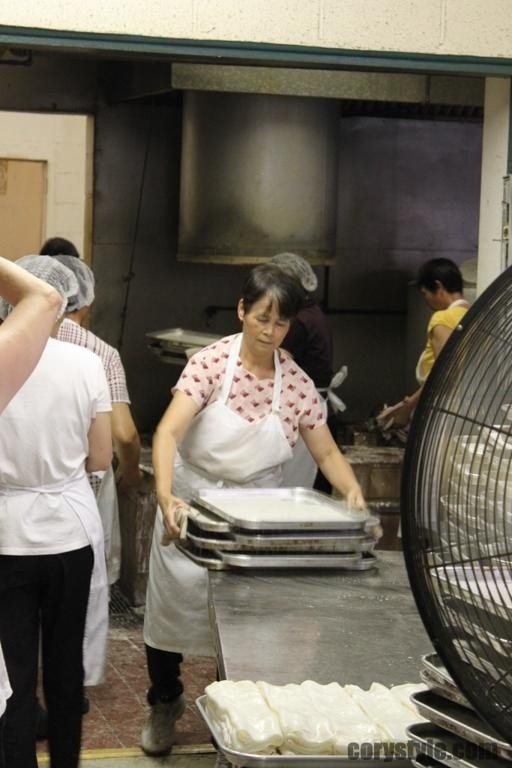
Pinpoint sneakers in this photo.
[140,694,185,757]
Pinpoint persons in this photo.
[39,237,81,257]
[375,257,473,437]
[4,256,114,766]
[45,258,140,716]
[267,249,355,494]
[144,260,386,756]
[2,257,61,724]
